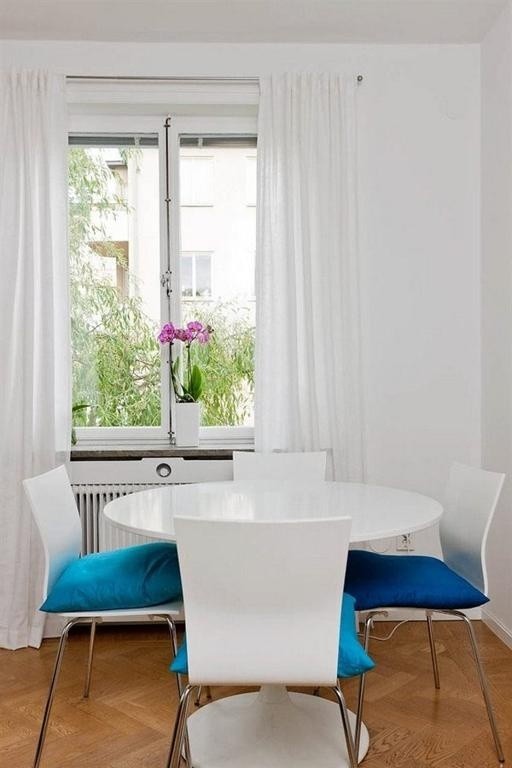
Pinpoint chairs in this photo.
[163,518,376,768]
[351,464,508,764]
[23,467,186,768]
[232,448,327,484]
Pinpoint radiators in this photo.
[63,482,184,558]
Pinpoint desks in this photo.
[96,483,445,768]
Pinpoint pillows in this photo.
[169,590,372,686]
[341,551,490,614]
[40,542,181,617]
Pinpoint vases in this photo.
[172,403,201,448]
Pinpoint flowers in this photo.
[157,320,215,403]
